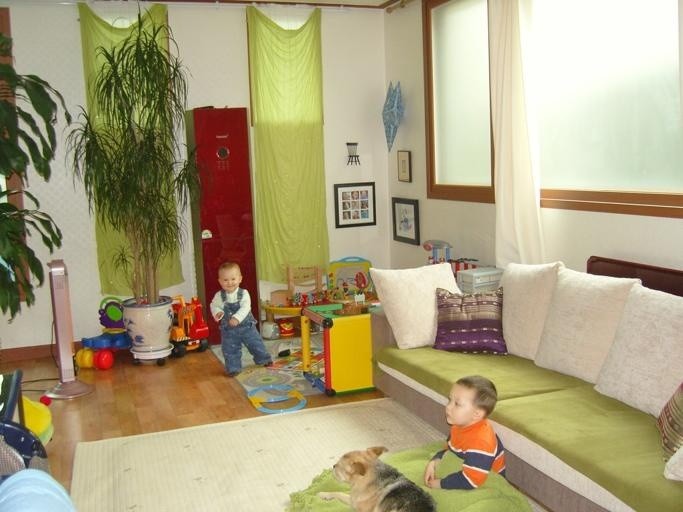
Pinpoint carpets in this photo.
[210,331,325,403]
[67,393,452,512]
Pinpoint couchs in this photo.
[368,304,682,512]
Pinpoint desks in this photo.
[1,367,27,428]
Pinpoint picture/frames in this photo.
[397,150,412,183]
[333,180,377,229]
[391,197,422,247]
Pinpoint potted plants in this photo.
[60,4,213,363]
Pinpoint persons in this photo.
[210,263,273,377]
[424,375,506,489]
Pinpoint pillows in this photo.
[654,382,683,465]
[591,281,683,419]
[531,264,643,388]
[497,260,564,362]
[369,260,462,351]
[663,445,682,483]
[434,284,508,357]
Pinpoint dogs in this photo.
[315,446,437,512]
